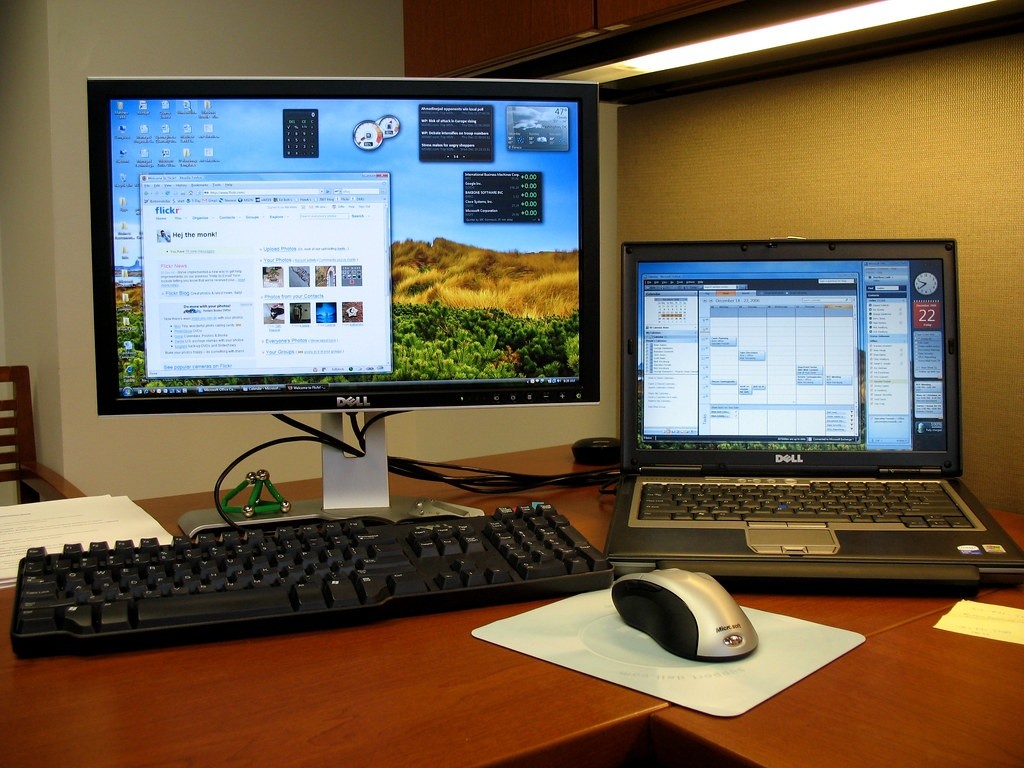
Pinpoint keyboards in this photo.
[8,504,616,659]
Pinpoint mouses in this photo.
[611,567,759,662]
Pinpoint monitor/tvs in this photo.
[86,75,601,539]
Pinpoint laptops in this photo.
[605,238,1024,590]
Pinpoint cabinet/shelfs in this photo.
[402,0,741,78]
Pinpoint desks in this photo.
[0,444,1024,768]
